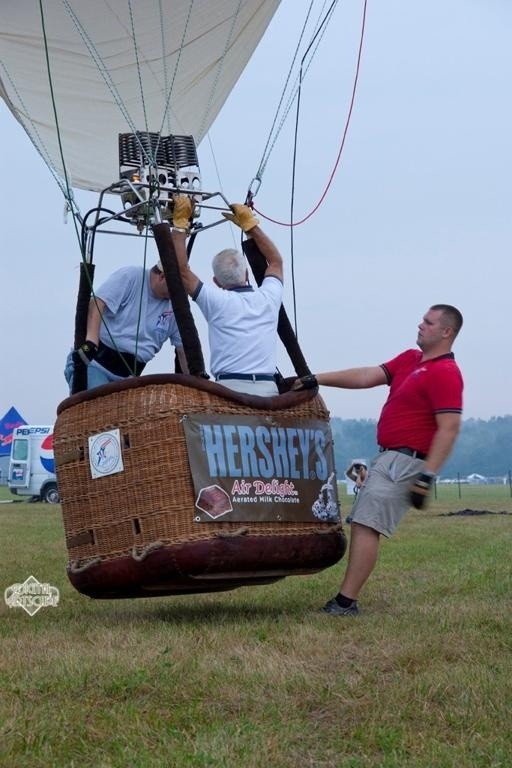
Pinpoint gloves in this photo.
[411,472,436,509]
[291,375,318,391]
[173,196,192,229]
[72,341,98,369]
[221,203,259,233]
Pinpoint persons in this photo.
[346,462,369,492]
[290,305,462,617]
[173,194,285,398]
[64,258,191,397]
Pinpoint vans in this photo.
[8,425,60,505]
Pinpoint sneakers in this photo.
[319,598,358,615]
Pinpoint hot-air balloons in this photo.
[0,0,367,602]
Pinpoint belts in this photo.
[380,446,427,460]
[215,374,275,381]
